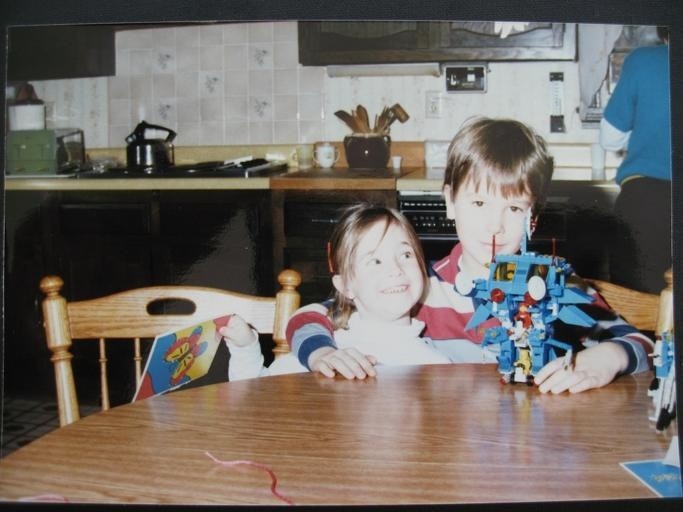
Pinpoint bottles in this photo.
[654,266,673,340]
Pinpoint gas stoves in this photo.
[76,158,289,177]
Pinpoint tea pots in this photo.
[126,119,176,173]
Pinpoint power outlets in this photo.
[424,92,443,120]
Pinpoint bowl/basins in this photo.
[343,135,390,169]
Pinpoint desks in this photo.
[1,362,677,506]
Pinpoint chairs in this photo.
[38,268,301,429]
[577,264,673,341]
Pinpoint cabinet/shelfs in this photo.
[296,19,577,66]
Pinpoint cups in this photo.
[291,146,315,170]
[8,100,45,130]
[392,156,402,168]
[313,143,340,169]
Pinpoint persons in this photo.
[282,113,654,395]
[218,201,500,382]
[601,24,671,294]
